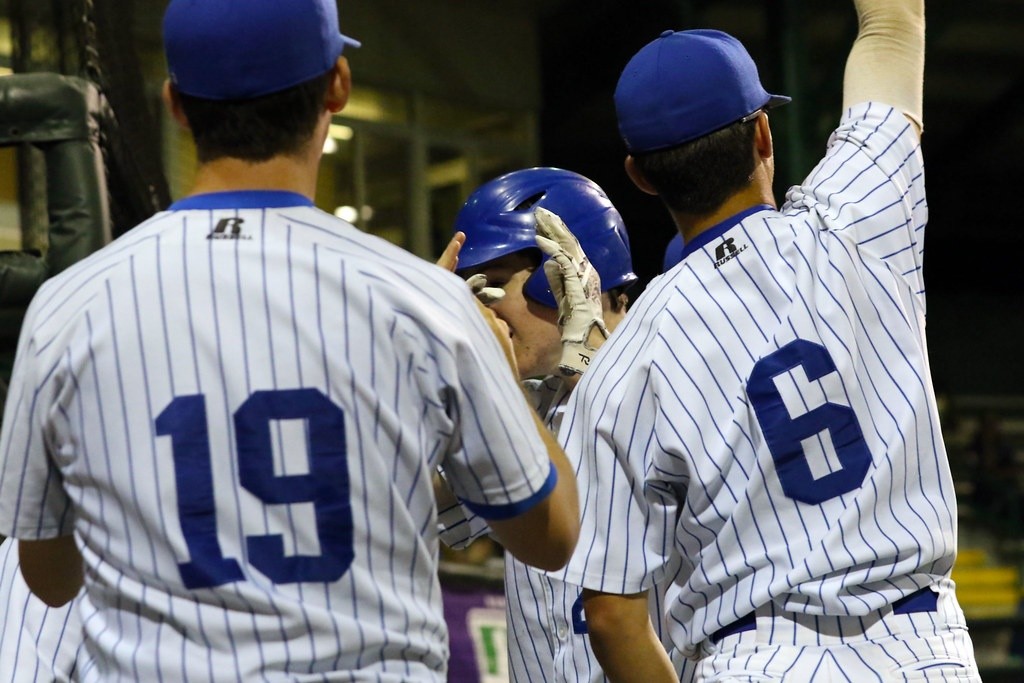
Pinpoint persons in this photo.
[428,166,640,683]
[528,0,983,683]
[0,0,579,683]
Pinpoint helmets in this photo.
[449,167,637,310]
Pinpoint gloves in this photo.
[464,271,505,304]
[531,207,611,377]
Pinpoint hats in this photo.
[613,28,792,154]
[163,0,362,101]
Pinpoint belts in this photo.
[709,582,938,642]
